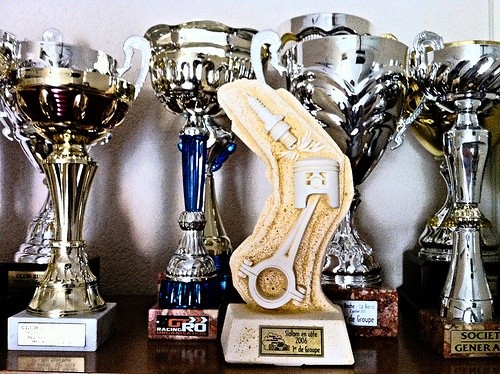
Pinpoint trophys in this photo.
[0,27,151,311]
[383,35,500,303]
[404,32,500,360]
[272,12,397,46]
[247,28,443,339]
[0,49,135,354]
[143,20,269,343]
[217,79,356,368]
